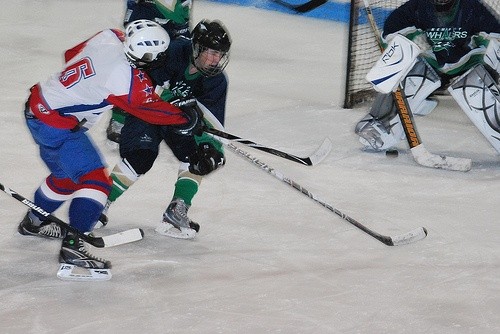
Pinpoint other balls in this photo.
[385,149,398,156]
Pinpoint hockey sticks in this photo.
[212,135,428,247]
[200,126,332,168]
[362,0,473,173]
[1,184,145,248]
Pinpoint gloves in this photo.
[188,142,225,176]
[169,104,204,137]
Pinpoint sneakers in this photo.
[93,199,113,228]
[105,118,124,151]
[154,198,199,239]
[56,226,113,281]
[16,208,66,240]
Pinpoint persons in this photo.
[23,18,205,271]
[361,0,500,155]
[104,0,195,147]
[90,18,232,233]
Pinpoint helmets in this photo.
[188,18,231,78]
[122,20,171,61]
